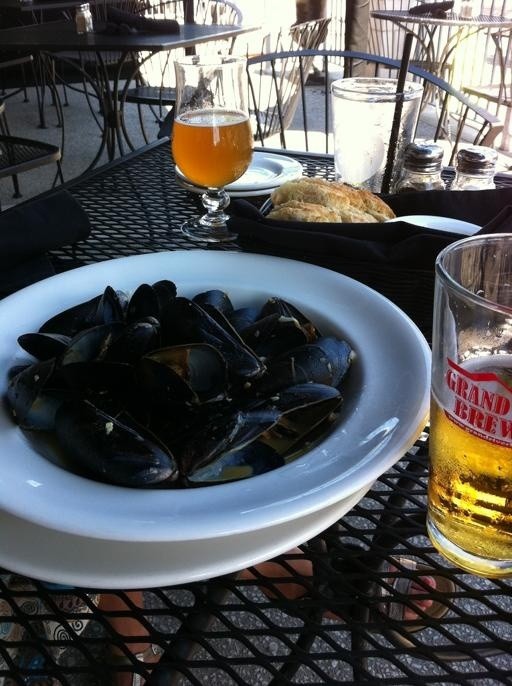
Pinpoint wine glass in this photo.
[170,55,255,242]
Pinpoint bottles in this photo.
[458,2,472,20]
[396,142,498,194]
[75,2,93,35]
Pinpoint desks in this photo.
[370,9,510,154]
[2,22,263,181]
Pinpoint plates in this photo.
[173,152,303,200]
[0,249,434,589]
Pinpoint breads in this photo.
[265,176,397,226]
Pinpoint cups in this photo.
[426,229,512,578]
[331,78,425,193]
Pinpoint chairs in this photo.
[1,1,96,130]
[460,28,511,155]
[103,1,243,145]
[1,130,68,196]
[156,14,333,151]
[244,49,504,172]
